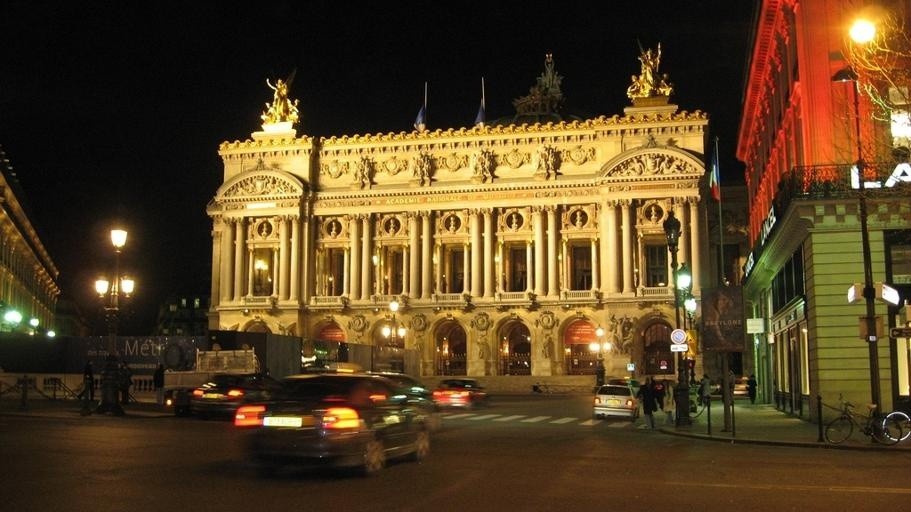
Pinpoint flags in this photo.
[414,105,426,134]
[474,106,485,128]
[708,151,721,202]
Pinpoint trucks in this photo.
[162,349,261,416]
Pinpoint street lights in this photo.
[662,211,700,427]
[589,327,612,393]
[96,221,135,414]
[834,18,884,412]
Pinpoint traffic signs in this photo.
[382,302,406,370]
[847,282,863,304]
[873,282,900,307]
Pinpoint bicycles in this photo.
[826,394,910,446]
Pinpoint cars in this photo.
[593,378,676,422]
[432,378,492,411]
[173,363,431,474]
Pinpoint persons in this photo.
[634,377,674,430]
[698,370,736,406]
[260,79,301,123]
[83,359,95,401]
[153,364,164,405]
[626,42,674,99]
[747,374,757,404]
[119,361,133,404]
